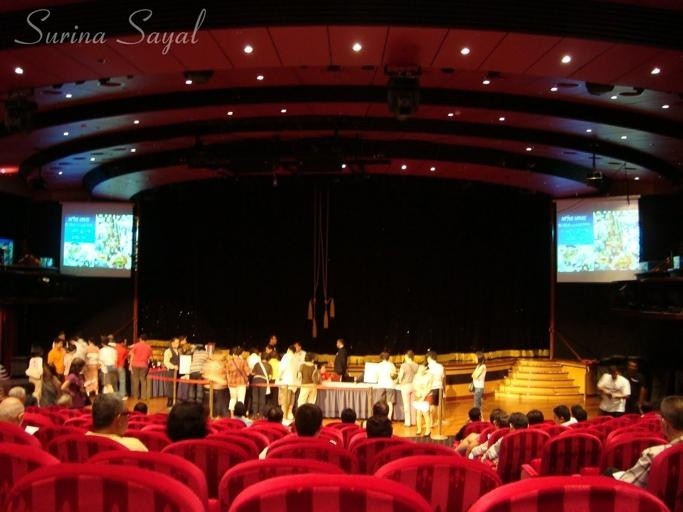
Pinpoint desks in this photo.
[146,366,403,421]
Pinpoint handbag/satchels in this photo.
[265,385,271,395]
[84,391,93,407]
[468,382,476,393]
[412,399,431,413]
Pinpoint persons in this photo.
[1,331,586,472]
[596,365,632,417]
[621,355,649,413]
[601,395,683,488]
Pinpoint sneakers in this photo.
[121,396,129,400]
